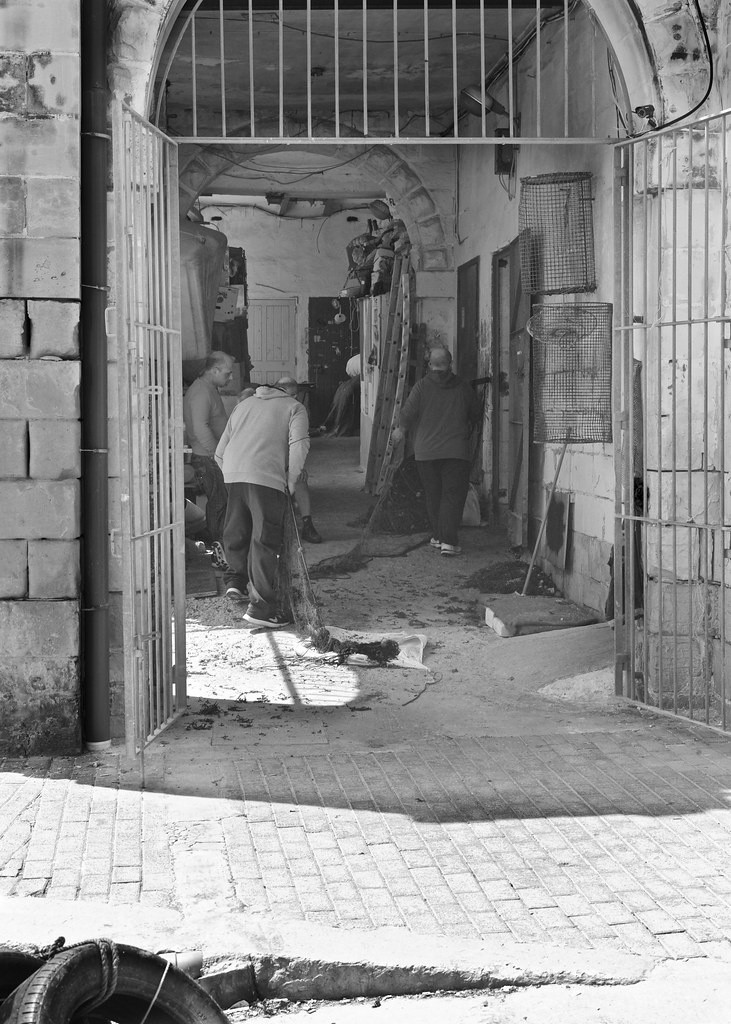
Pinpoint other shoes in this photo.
[429,538,461,556]
[209,541,234,571]
[303,521,320,543]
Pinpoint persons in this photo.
[213,376,315,629]
[236,385,324,544]
[182,350,238,570]
[391,348,485,556]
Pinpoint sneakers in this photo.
[226,581,248,600]
[242,603,290,628]
